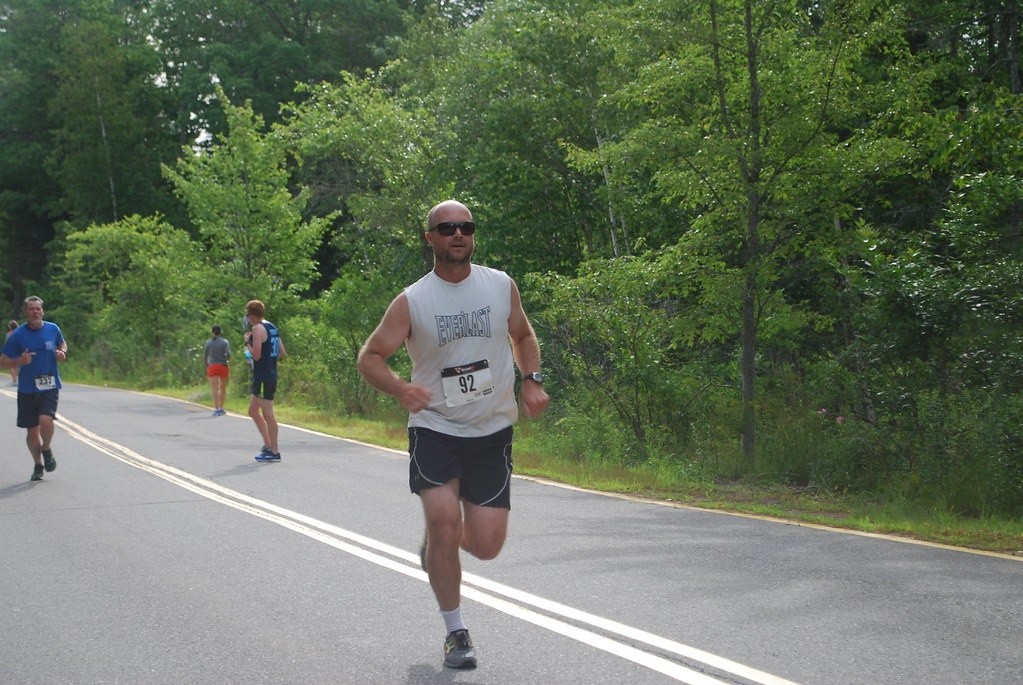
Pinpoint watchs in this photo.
[522,371,545,384]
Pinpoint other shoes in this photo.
[212,410,221,416]
[220,409,225,415]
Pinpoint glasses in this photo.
[428,222,475,237]
[246,313,255,318]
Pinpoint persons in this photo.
[204,326,231,416]
[5,320,19,386]
[358,200,549,667]
[0,296,67,481]
[244,300,286,462]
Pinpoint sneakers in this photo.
[31,464,44,480]
[42,445,56,472]
[254,446,271,461]
[259,448,281,462]
[420,527,429,573]
[444,629,477,669]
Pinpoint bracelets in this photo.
[60,349,67,356]
[245,341,252,347]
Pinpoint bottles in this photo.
[244,346,253,364]
